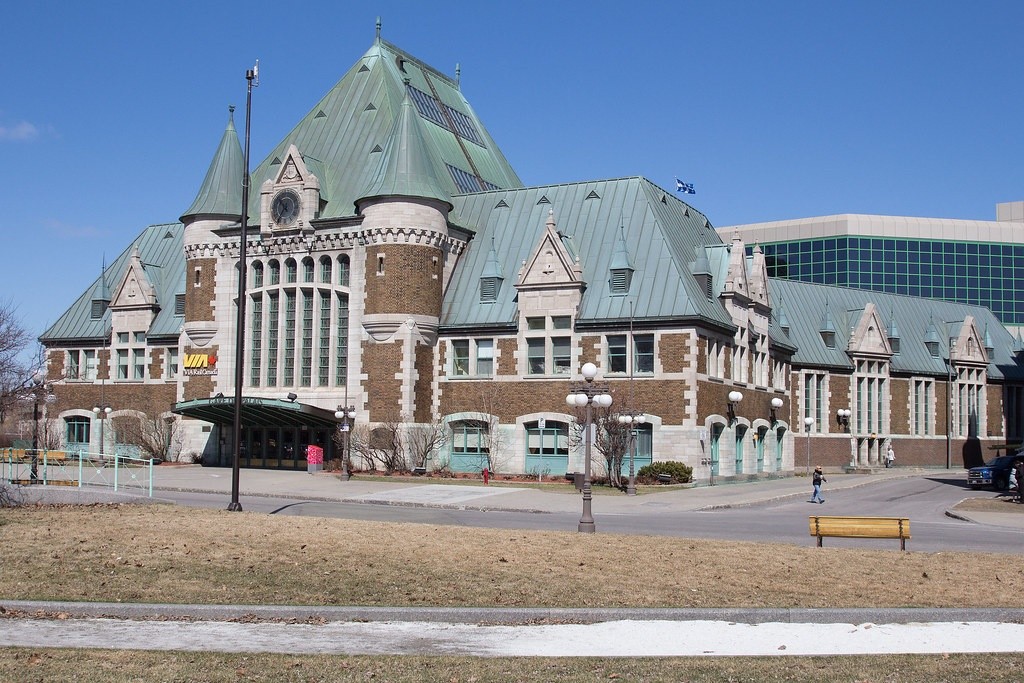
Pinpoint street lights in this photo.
[22,373,58,484]
[563,360,614,535]
[617,410,646,494]
[947,336,960,470]
[333,405,357,482]
[226,58,263,513]
[803,417,815,478]
[92,403,113,468]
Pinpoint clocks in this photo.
[270,188,301,228]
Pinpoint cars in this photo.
[966,452,1024,491]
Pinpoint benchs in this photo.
[1009,489,1018,503]
[39,451,65,465]
[4,449,25,463]
[808,517,912,551]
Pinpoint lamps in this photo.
[837,408,851,424]
[770,398,783,420]
[726,391,743,416]
[287,393,297,402]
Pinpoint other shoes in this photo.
[811,501,817,503]
[820,500,825,504]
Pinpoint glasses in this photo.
[819,468,822,469]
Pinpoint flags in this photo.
[677,178,696,194]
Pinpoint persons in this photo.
[810,466,828,504]
[1014,460,1024,504]
[888,448,896,468]
[1009,468,1019,491]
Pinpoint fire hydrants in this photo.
[482,467,489,485]
